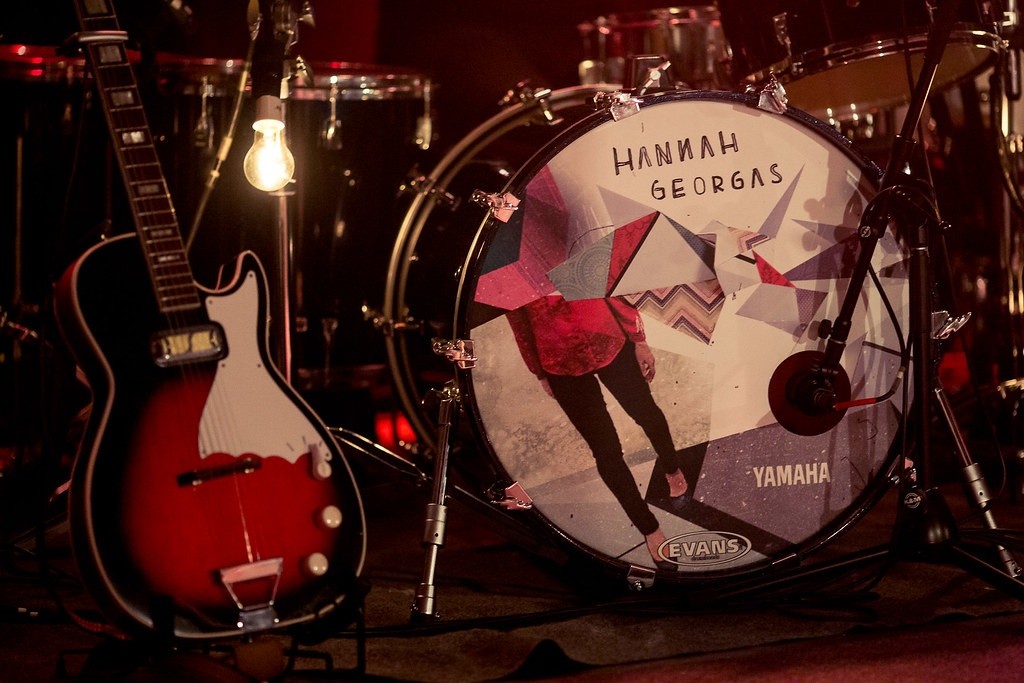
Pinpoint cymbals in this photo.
[0,43,140,83]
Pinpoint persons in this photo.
[504,295,690,576]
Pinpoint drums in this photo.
[740,27,1004,170]
[577,4,735,92]
[158,58,432,201]
[378,81,945,584]
[55,0,367,643]
